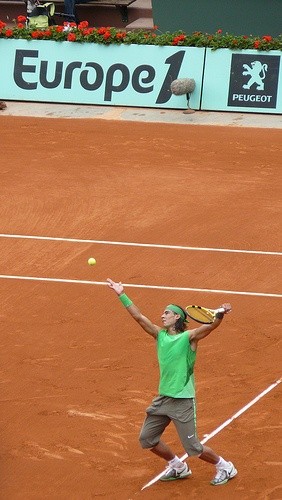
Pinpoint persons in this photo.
[105,276,239,485]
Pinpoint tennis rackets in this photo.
[184,305,226,324]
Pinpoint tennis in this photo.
[88,257,96,266]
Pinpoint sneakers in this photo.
[159,462,192,481]
[210,461,237,485]
